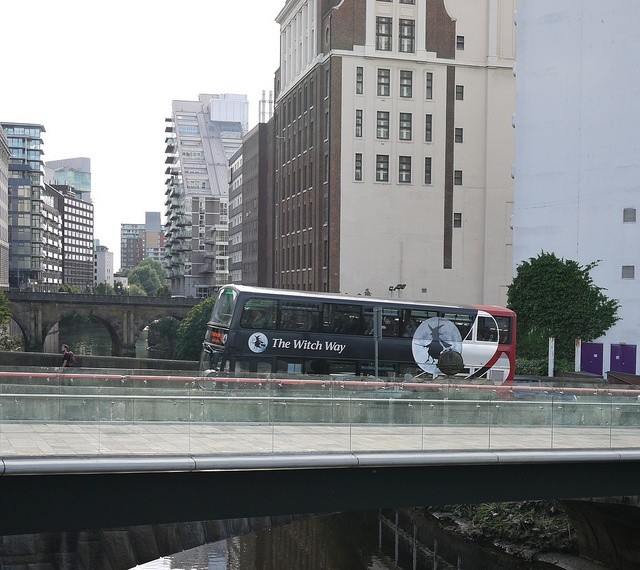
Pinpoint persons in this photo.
[59,344,77,386]
[252,311,266,329]
[382,317,393,337]
[401,318,417,338]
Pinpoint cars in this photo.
[513,375,579,413]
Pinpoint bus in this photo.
[197,282,518,406]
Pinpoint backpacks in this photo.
[63,351,76,366]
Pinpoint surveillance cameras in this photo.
[396,284,406,289]
